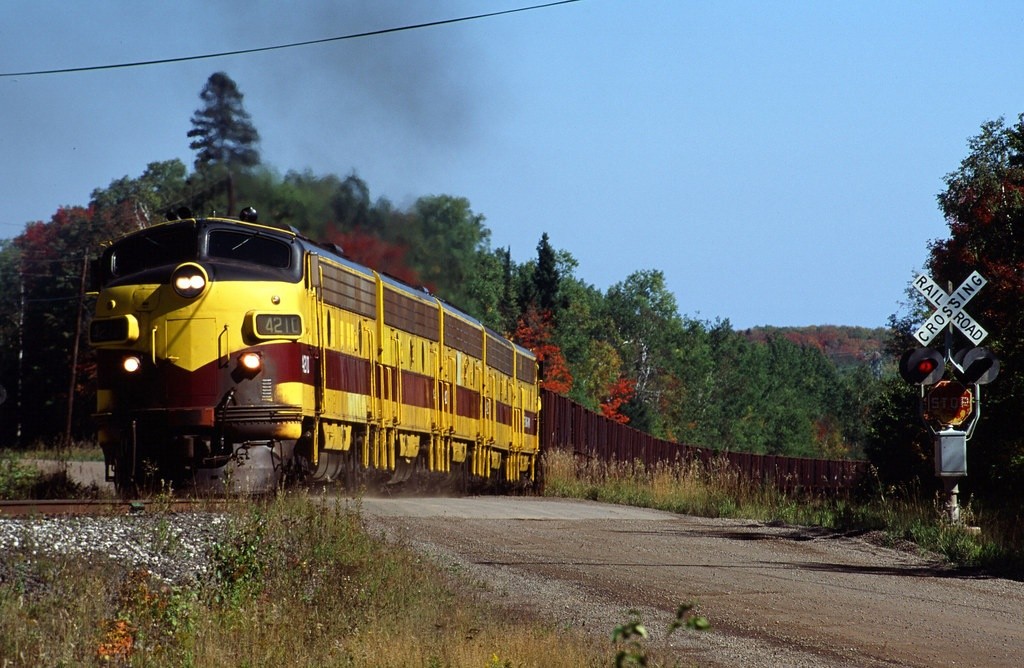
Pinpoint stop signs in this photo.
[922,381,973,427]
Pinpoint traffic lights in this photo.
[964,348,1000,384]
[901,348,946,387]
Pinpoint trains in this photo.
[83,204,879,501]
